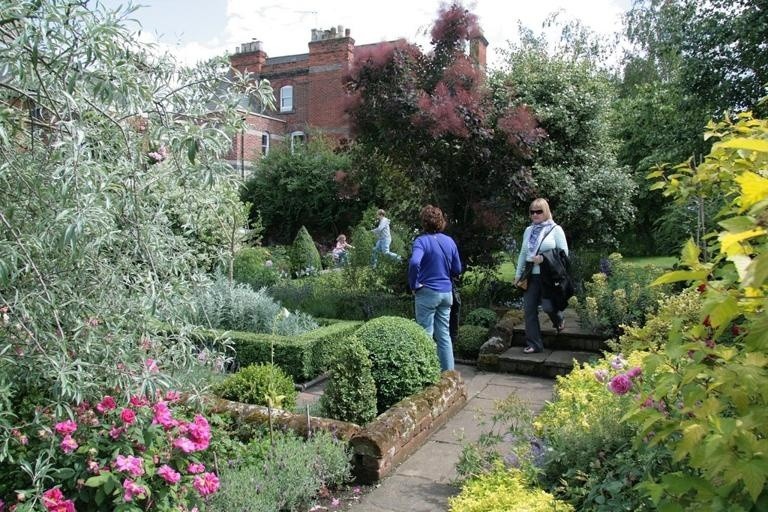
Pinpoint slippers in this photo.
[558,319,565,331]
[524,347,535,354]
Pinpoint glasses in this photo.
[529,210,542,215]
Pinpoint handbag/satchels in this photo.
[449,291,460,340]
[516,262,532,291]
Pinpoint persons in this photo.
[408,205,462,373]
[514,197,568,354]
[370,210,402,269]
[333,235,354,263]
